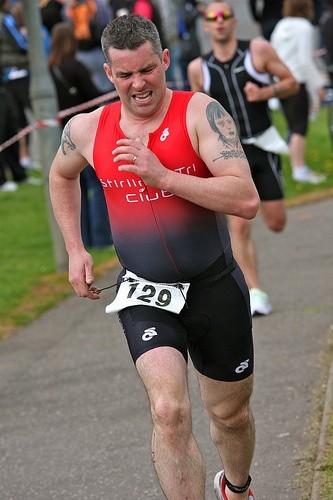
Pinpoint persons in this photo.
[270,0,327,183]
[187,3,299,318]
[48,15,260,500]
[0,1,208,192]
[250,0,332,89]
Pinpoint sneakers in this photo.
[26,178,44,184]
[212,472,255,500]
[1,182,18,193]
[290,167,328,185]
[250,289,274,318]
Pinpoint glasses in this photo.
[203,10,234,24]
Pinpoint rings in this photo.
[132,155,138,164]
